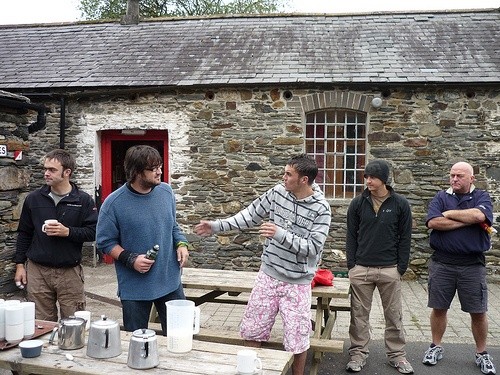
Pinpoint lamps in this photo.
[372,96,382,108]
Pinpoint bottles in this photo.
[479,220,498,237]
[139,244,159,273]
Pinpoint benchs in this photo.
[117,318,345,354]
[183,292,352,311]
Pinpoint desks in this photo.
[0,330,293,375]
[148,268,352,375]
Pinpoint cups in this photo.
[73,310,91,330]
[237,350,262,375]
[42,219,59,236]
[0,299,36,342]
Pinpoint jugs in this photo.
[165,300,200,354]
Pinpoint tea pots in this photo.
[49,315,88,350]
[86,314,123,358]
[127,328,159,369]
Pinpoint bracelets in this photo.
[119,249,138,270]
[177,241,188,248]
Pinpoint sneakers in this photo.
[387,356,414,374]
[346,358,366,373]
[475,352,496,375]
[422,344,444,365]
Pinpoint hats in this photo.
[364,161,389,184]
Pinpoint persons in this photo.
[345,159,415,375]
[95,146,190,338]
[424,161,497,375]
[195,155,333,375]
[15,149,98,323]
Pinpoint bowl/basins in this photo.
[19,339,42,357]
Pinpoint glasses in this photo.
[143,162,163,173]
